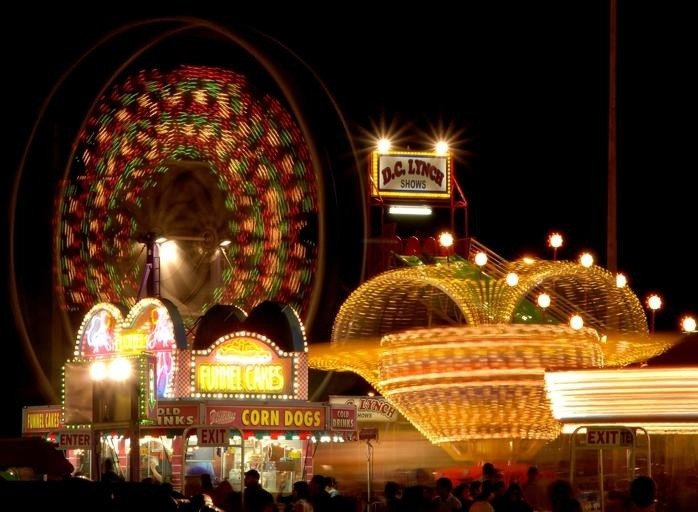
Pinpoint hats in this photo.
[243,470,260,480]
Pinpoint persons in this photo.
[102,457,697,511]
